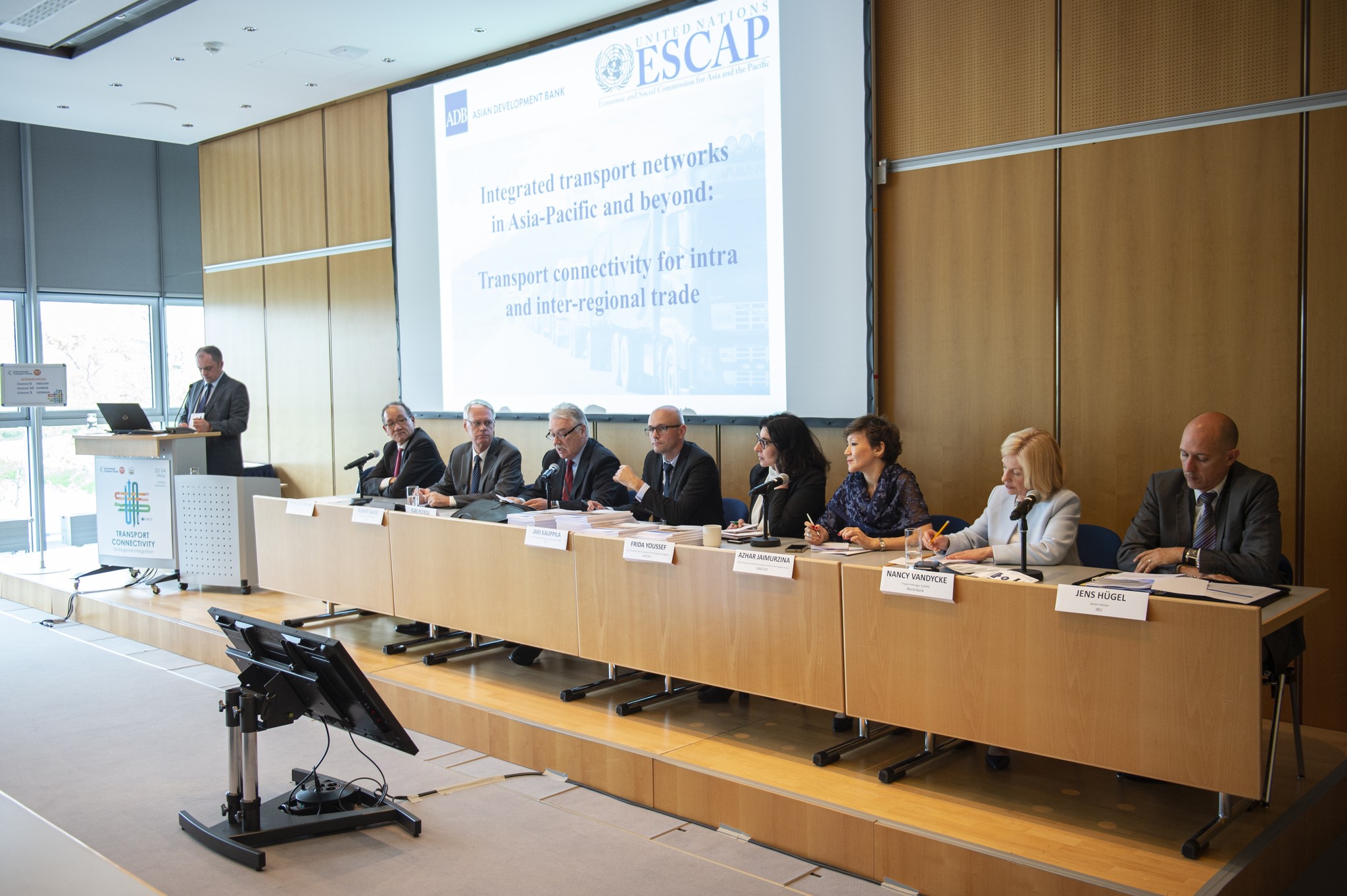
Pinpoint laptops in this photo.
[96,403,164,434]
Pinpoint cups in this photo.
[703,525,722,548]
[904,528,922,568]
[191,467,199,475]
[87,413,98,434]
[406,486,419,506]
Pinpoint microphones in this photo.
[344,450,380,470]
[166,384,197,433]
[540,463,560,481]
[749,473,790,497]
[1009,490,1041,521]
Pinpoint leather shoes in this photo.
[509,645,543,665]
[504,642,520,648]
[462,633,479,638]
[395,620,450,635]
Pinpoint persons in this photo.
[803,413,934,733]
[1117,411,1282,783]
[505,402,630,667]
[359,401,447,615]
[922,427,1082,772]
[176,346,250,477]
[394,399,526,636]
[587,405,725,680]
[698,412,832,705]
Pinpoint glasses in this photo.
[645,425,680,436]
[757,433,773,449]
[545,424,581,440]
[385,415,412,428]
[464,418,495,428]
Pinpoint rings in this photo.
[964,554,966,556]
[1148,558,1152,563]
[806,533,809,538]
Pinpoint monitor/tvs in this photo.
[206,606,419,756]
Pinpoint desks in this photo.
[253,489,1329,858]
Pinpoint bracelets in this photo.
[1182,547,1189,564]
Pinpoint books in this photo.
[721,523,764,539]
[810,542,876,556]
[1085,572,1186,593]
[506,509,703,546]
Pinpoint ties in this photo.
[664,462,674,497]
[470,455,482,493]
[563,460,575,500]
[197,383,211,413]
[394,448,404,477]
[1191,492,1216,551]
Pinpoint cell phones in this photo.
[913,561,939,571]
[727,537,758,544]
[785,544,809,552]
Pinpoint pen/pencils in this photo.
[928,520,950,546]
[806,513,821,538]
[422,494,425,497]
[581,499,597,509]
[1091,574,1111,581]
[730,521,757,528]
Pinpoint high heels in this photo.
[697,686,750,704]
[985,745,1010,769]
[832,712,854,732]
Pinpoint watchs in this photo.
[877,537,886,551]
[550,500,559,509]
[1186,547,1198,566]
